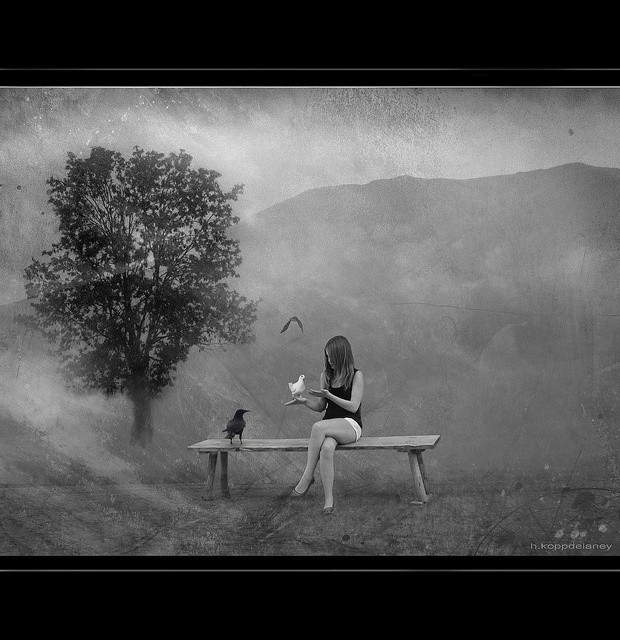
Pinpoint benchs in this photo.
[187,435,438,504]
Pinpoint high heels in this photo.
[290,476,314,498]
[323,497,334,514]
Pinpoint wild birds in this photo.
[279,315,303,334]
[222,409,251,444]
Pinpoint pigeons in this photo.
[288,374,306,400]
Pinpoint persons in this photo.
[284,335,364,515]
